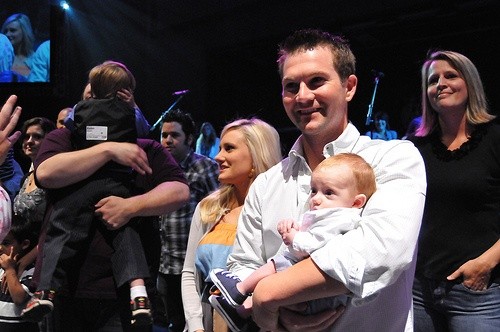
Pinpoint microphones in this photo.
[172,89,192,95]
[371,69,384,77]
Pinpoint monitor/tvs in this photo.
[0,0,57,88]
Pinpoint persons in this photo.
[181,118,283,332]
[33,81,190,332]
[196,122,221,160]
[0,13,50,82]
[208,153,376,332]
[365,111,400,142]
[156,110,220,332]
[0,94,74,332]
[403,50,500,332]
[20,61,155,325]
[227,29,427,332]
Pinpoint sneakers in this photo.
[21,290,55,323]
[210,268,248,306]
[208,295,246,332]
[130,296,154,328]
